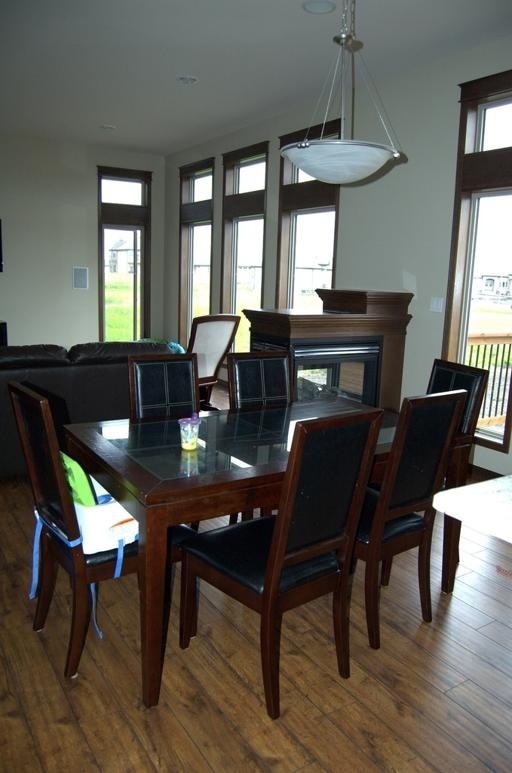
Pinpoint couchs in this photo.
[0,339,187,479]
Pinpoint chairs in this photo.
[425,357,490,466]
[178,409,384,720]
[124,353,237,533]
[225,346,293,518]
[6,383,197,676]
[184,313,240,395]
[352,388,465,650]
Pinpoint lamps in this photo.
[279,1,401,187]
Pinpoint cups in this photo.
[178,452,200,479]
[178,413,202,451]
